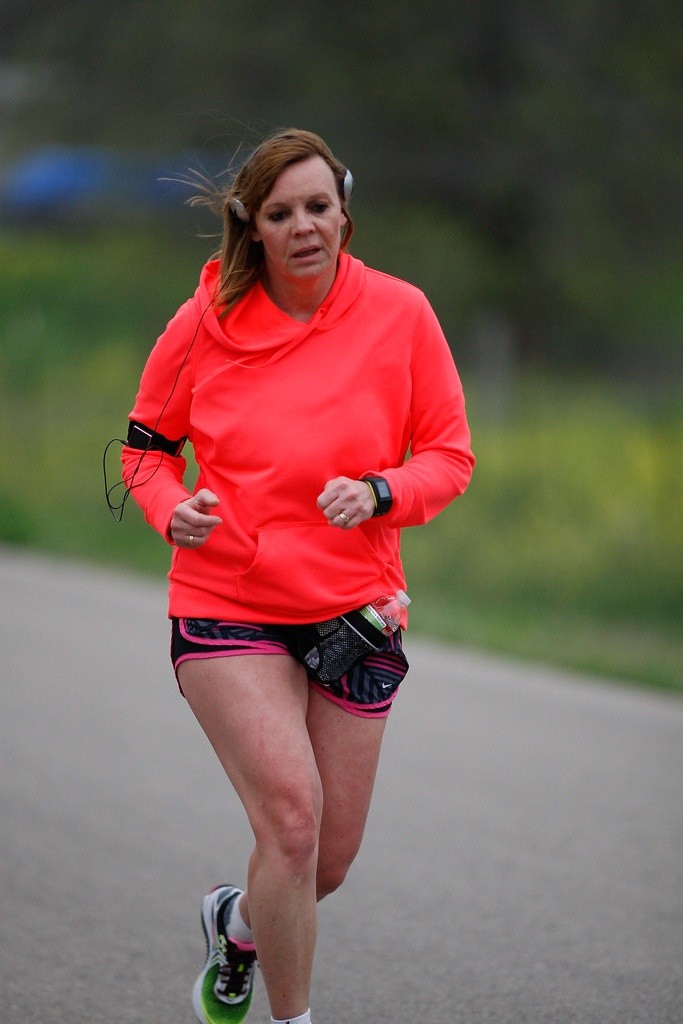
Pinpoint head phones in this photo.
[230,134,354,221]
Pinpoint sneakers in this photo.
[190,882,259,1024]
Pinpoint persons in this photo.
[123,128,479,1024]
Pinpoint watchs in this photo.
[359,476,394,518]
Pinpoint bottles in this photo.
[303,590,410,686]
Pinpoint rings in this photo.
[190,536,194,545]
[340,514,351,524]
